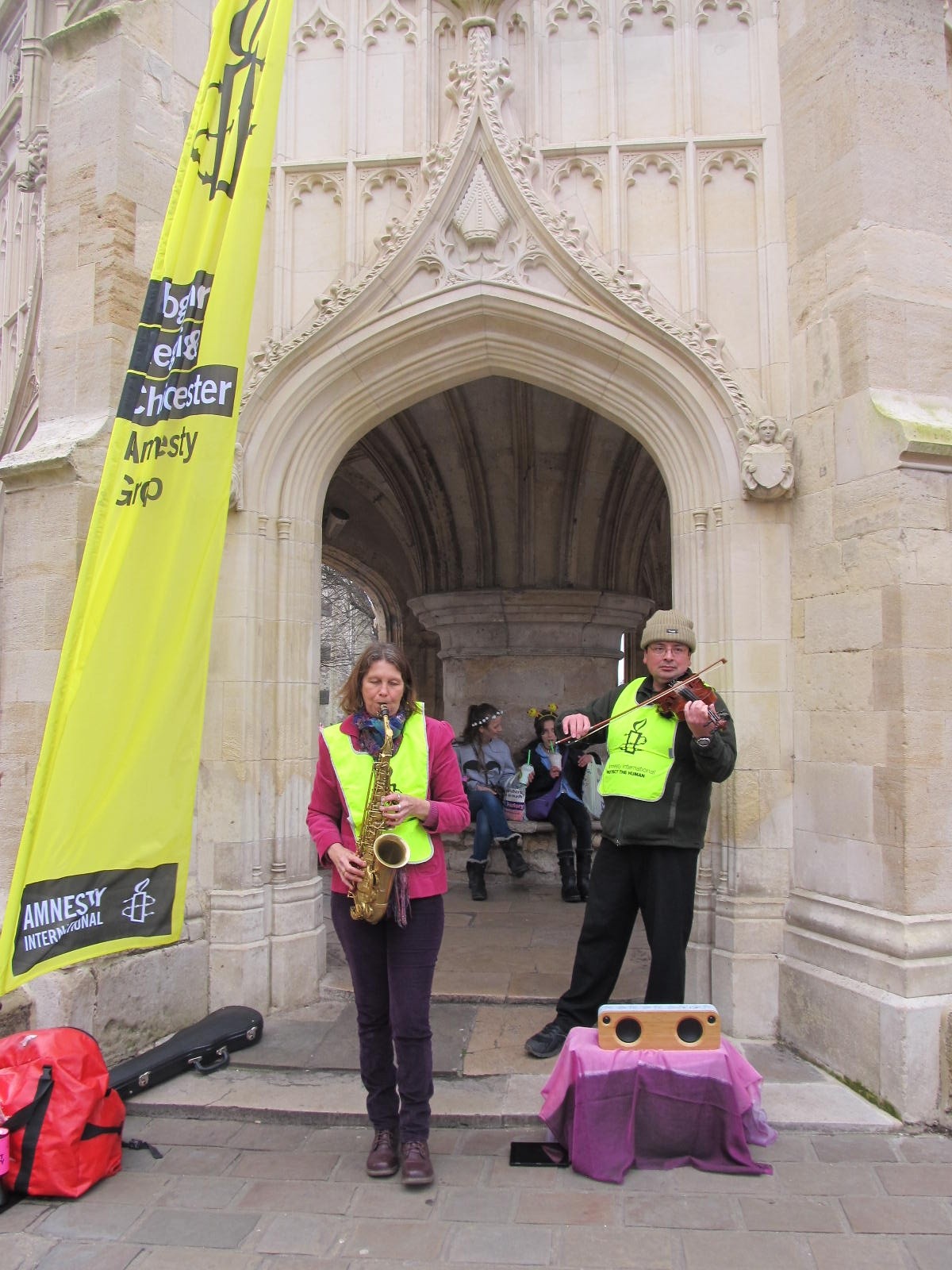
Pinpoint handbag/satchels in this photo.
[0,1027,126,1198]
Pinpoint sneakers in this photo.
[525,1022,567,1057]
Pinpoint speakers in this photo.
[598,1003,721,1050]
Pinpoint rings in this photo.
[397,794,401,801]
[341,871,347,875]
[397,805,402,813]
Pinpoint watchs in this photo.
[691,732,714,748]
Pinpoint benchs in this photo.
[438,821,603,886]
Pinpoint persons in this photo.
[306,643,471,1184]
[525,610,738,1059]
[450,704,594,901]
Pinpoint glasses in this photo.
[649,645,685,655]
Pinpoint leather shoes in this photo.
[401,1141,434,1185]
[365,1130,399,1177]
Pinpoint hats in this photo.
[640,609,696,653]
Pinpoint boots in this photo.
[575,846,594,901]
[494,833,530,876]
[466,855,488,900]
[557,849,582,903]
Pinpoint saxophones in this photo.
[347,702,410,927]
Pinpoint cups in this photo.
[549,752,562,771]
[519,763,534,785]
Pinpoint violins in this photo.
[653,673,731,736]
